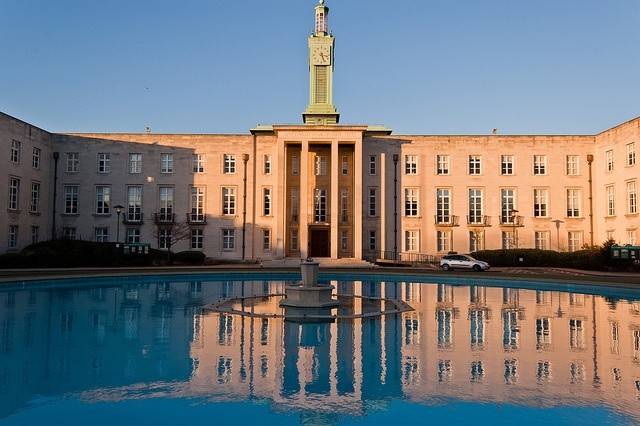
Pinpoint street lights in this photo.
[114,204,126,266]
[509,209,520,268]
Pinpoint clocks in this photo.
[312,45,330,65]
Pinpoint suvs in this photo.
[440,254,490,272]
